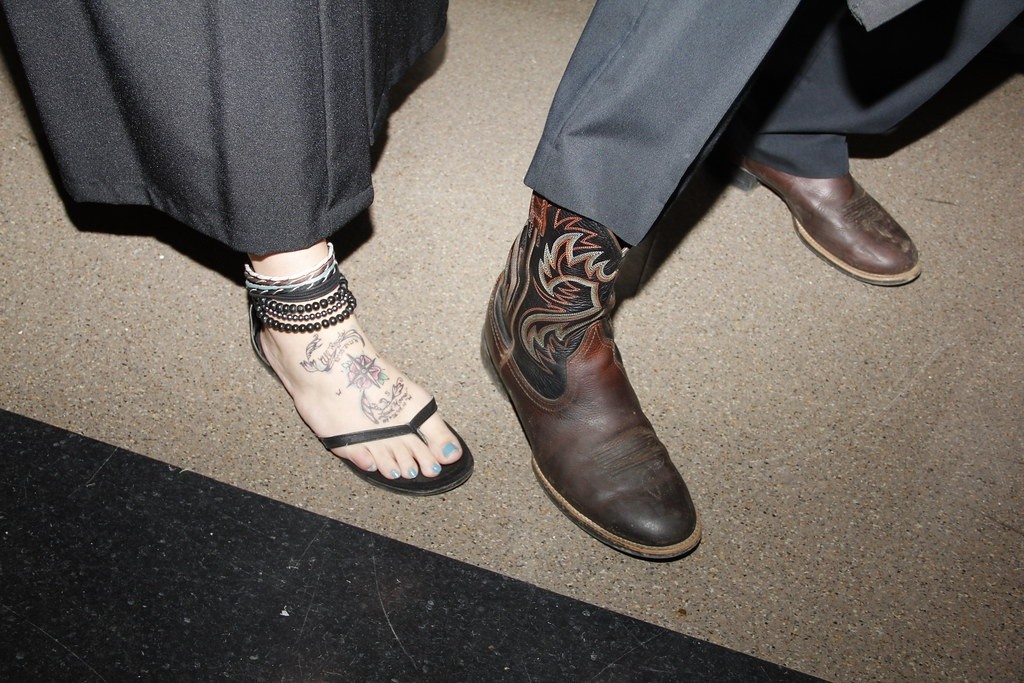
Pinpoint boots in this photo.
[729,156,922,289]
[481,190,703,560]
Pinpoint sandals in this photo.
[245,241,476,496]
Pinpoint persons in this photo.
[481,0,1024,563]
[0,2,475,497]
